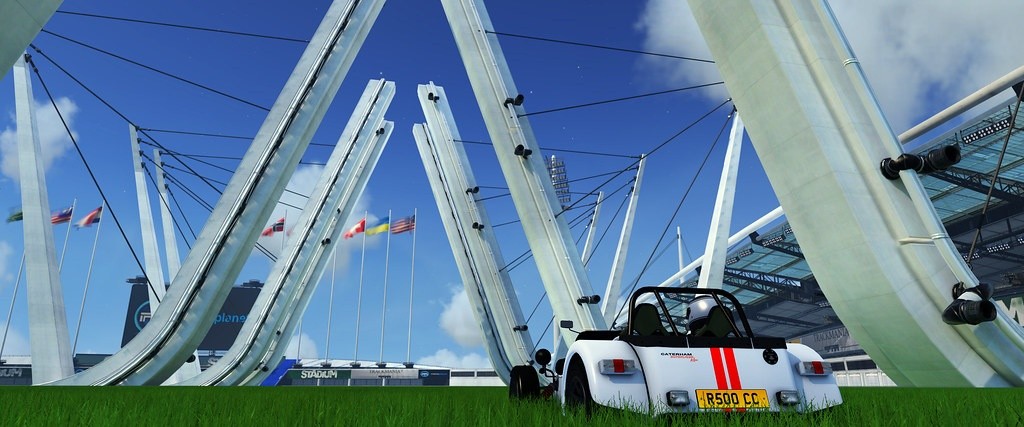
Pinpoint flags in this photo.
[262,217,283,235]
[76,206,102,228]
[50,206,72,223]
[391,215,415,233]
[7,211,23,222]
[366,217,388,235]
[345,218,365,238]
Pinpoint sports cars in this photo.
[558,286,845,424]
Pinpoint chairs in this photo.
[620,303,670,336]
[695,306,736,336]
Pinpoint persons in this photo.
[686,295,728,338]
